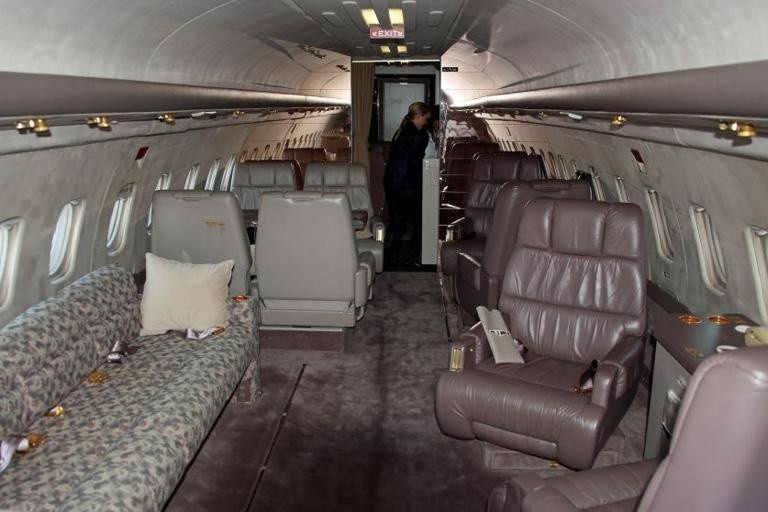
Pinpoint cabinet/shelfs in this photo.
[636,313,752,461]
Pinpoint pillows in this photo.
[134,251,235,337]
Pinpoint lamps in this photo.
[716,117,757,139]
[155,112,177,126]
[81,113,109,130]
[610,114,629,129]
[15,111,49,138]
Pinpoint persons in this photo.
[382,101,439,266]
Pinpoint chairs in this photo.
[281,147,325,160]
[438,192,649,472]
[150,189,256,298]
[229,161,387,326]
[485,343,767,510]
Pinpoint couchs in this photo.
[439,132,595,343]
[1,258,260,511]
